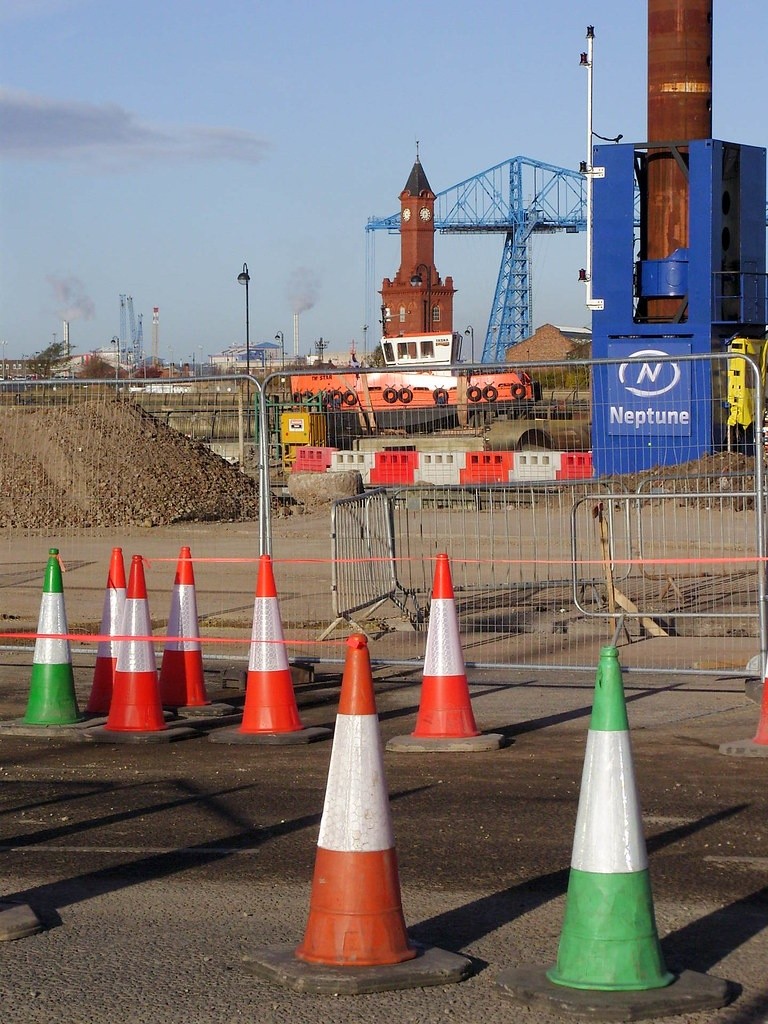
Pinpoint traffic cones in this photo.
[0,547,105,737]
[241,633,473,993]
[81,547,203,743]
[488,645,730,1018]
[207,554,333,745]
[156,545,237,716]
[717,655,768,759]
[385,554,506,753]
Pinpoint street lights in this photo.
[110,335,119,403]
[409,264,431,331]
[237,263,252,438]
[464,326,474,363]
[274,331,285,402]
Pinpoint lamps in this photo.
[578,269,587,282]
[579,161,587,173]
[587,27,595,37]
[579,51,589,66]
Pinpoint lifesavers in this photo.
[466,386,482,402]
[482,385,498,402]
[303,391,314,405]
[433,388,449,408]
[511,384,526,399]
[329,390,343,406]
[397,388,413,403]
[343,391,358,406]
[291,392,302,406]
[383,388,398,403]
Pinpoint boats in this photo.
[290,300,542,429]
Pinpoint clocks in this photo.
[402,208,411,222]
[418,206,432,222]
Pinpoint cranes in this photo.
[364,155,642,372]
